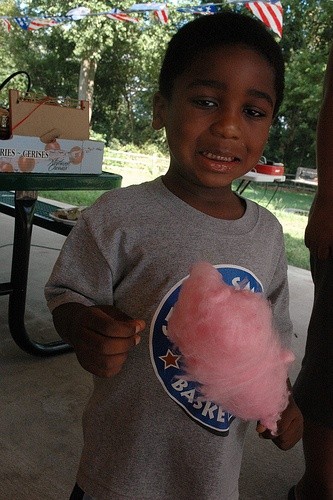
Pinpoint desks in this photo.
[0,170,123,356]
[236,172,286,208]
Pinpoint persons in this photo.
[44,14,305,500]
[293,39,333,499]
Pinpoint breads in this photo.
[55,207,83,220]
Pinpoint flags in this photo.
[2,0,283,38]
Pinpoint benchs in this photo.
[0,191,77,356]
[290,167,319,192]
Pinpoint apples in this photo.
[0,141,83,173]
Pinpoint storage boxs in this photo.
[256,164,285,175]
[0,134,105,174]
